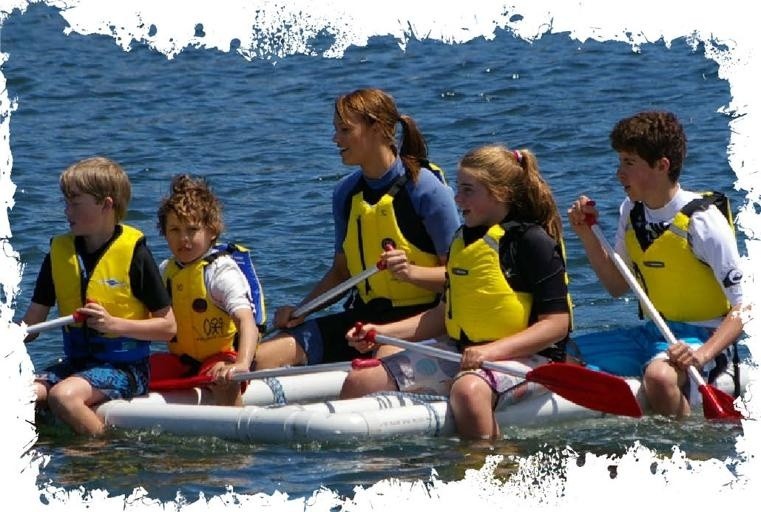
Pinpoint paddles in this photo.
[148,358,382,391]
[585,201,743,421]
[356,321,643,417]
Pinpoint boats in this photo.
[88,356,756,445]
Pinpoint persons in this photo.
[140,174,255,408]
[13,157,179,435]
[336,146,568,439]
[568,112,744,415]
[256,86,458,367]
